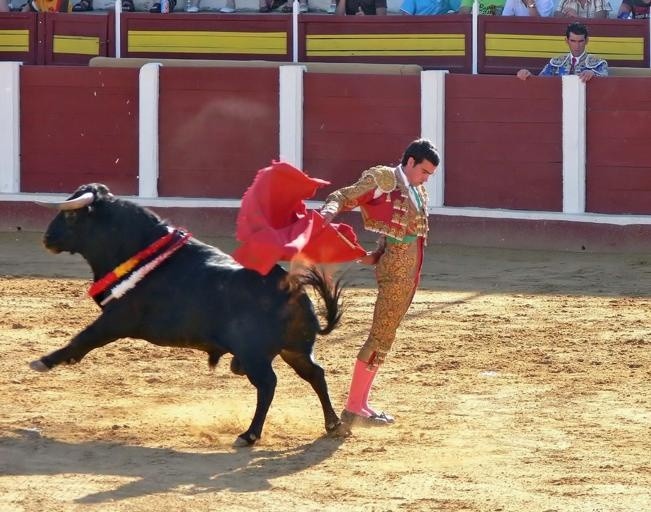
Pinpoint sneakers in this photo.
[300,3,309,11]
[328,4,336,13]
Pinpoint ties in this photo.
[569,58,576,74]
[411,186,422,210]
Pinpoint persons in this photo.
[320,140,440,428]
[517,22,608,83]
[2,0,176,12]
[470,0,651,20]
[260,0,462,16]
[185,0,236,13]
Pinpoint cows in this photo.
[29,182,355,449]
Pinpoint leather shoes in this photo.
[341,408,394,426]
[188,7,199,12]
[219,7,237,12]
[260,7,270,11]
[282,6,293,12]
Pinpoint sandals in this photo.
[150,0,176,12]
[121,0,134,12]
[72,1,93,12]
